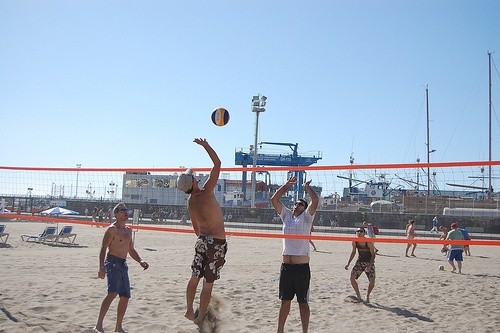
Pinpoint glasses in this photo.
[355,230,364,232]
[294,202,305,207]
[118,209,128,213]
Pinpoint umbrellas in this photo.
[41,205,79,235]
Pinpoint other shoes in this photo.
[93,326,104,333]
[113,328,126,333]
[411,254,416,257]
[374,250,378,253]
[405,255,409,257]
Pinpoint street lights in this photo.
[250,93,268,207]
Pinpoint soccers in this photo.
[438,265,445,270]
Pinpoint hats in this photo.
[450,223,456,227]
[177,168,193,192]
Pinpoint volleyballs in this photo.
[211,107,230,127]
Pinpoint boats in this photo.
[304,196,363,213]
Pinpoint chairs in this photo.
[21,226,57,243]
[40,226,77,245]
[0,225,10,246]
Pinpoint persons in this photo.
[343,227,376,303]
[363,221,379,253]
[94,202,149,333]
[176,138,228,324]
[269,177,319,333]
[438,226,450,249]
[431,217,439,233]
[447,223,471,274]
[16,208,21,215]
[140,208,188,224]
[225,210,236,222]
[405,219,417,257]
[309,239,316,252]
[318,215,340,229]
[31,205,41,215]
[85,205,113,223]
